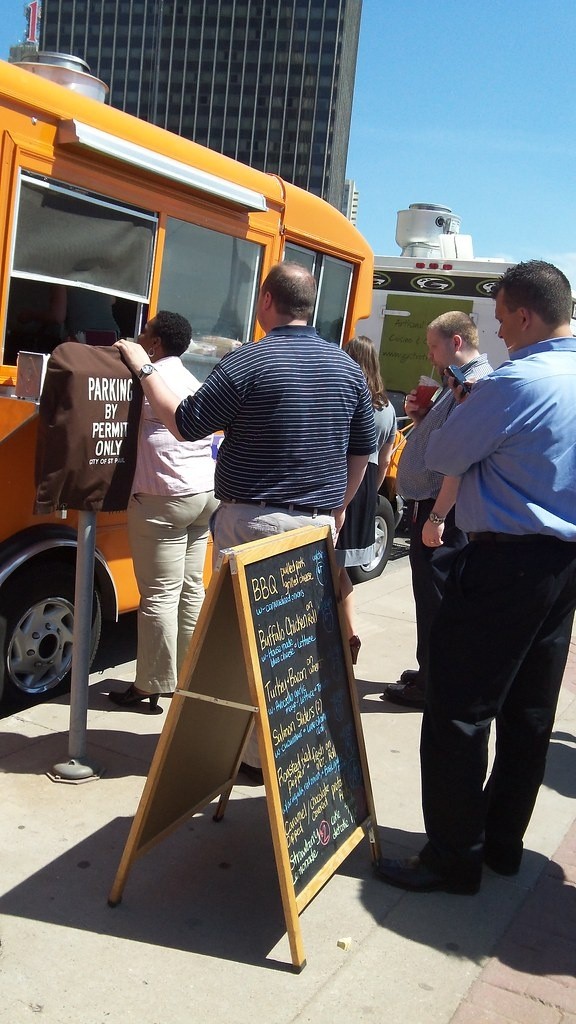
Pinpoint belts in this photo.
[467,531,543,546]
[228,498,336,520]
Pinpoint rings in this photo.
[431,542,434,544]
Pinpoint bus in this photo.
[354,203,575,438]
[0,52,416,709]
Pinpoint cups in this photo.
[15,351,43,398]
[0,384,15,397]
[412,374,440,408]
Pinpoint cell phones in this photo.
[447,365,471,396]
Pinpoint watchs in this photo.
[138,364,157,378]
[428,511,446,526]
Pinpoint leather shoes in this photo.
[385,669,428,705]
[373,855,482,895]
[479,845,520,876]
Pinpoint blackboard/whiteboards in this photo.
[134,524,376,914]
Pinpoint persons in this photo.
[369,262,576,896]
[384,311,494,708]
[342,335,397,663]
[108,311,220,711]
[113,262,379,786]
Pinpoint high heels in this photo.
[109,686,159,712]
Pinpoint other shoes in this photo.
[348,636,361,664]
